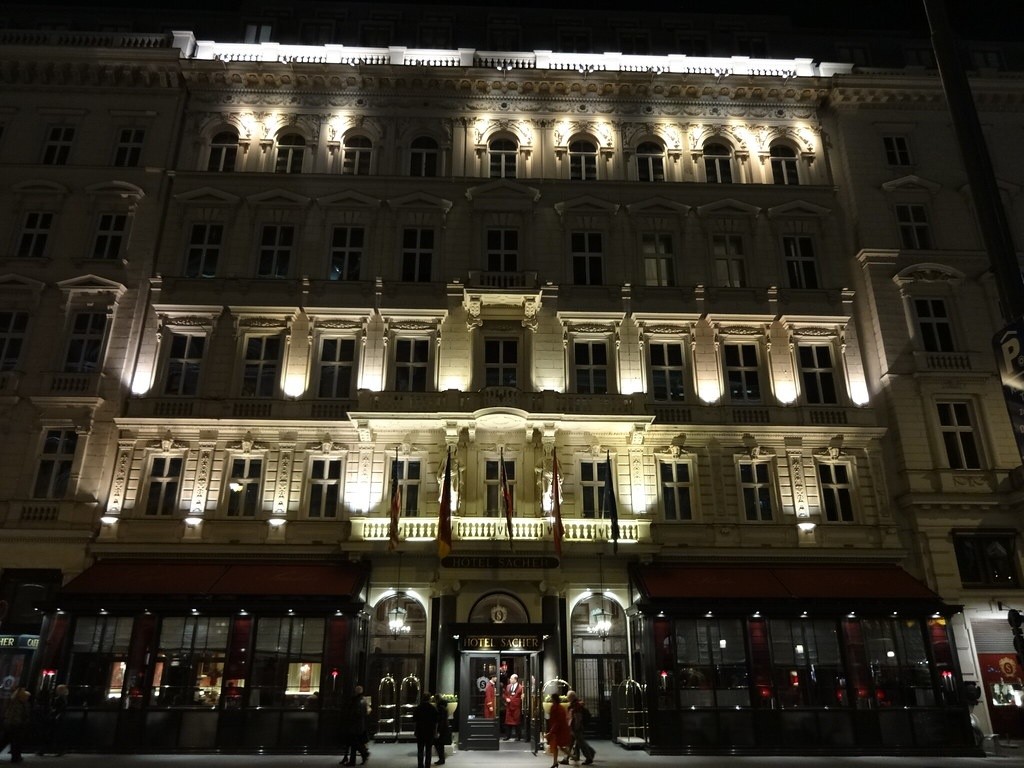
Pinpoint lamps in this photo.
[388,551,410,640]
[282,56,297,71]
[587,552,613,641]
[497,62,512,77]
[714,73,729,84]
[579,66,593,78]
[646,69,661,81]
[782,73,797,87]
[350,59,364,75]
[214,55,230,69]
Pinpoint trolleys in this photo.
[396,674,421,741]
[616,678,647,750]
[373,676,397,743]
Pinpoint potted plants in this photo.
[543,694,570,719]
[431,694,458,719]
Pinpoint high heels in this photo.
[550,764,558,768]
[574,755,579,761]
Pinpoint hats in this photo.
[547,694,561,702]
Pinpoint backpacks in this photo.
[581,708,592,727]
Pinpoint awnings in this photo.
[631,554,944,620]
[33,553,371,620]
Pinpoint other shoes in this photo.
[344,762,355,767]
[434,760,445,765]
[360,751,369,765]
[503,737,511,741]
[582,759,593,765]
[559,759,569,765]
[515,736,522,741]
[339,759,349,764]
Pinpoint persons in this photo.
[412,695,440,768]
[339,686,380,767]
[377,673,395,705]
[483,661,596,768]
[0,684,70,763]
[401,676,421,706]
[433,695,452,765]
